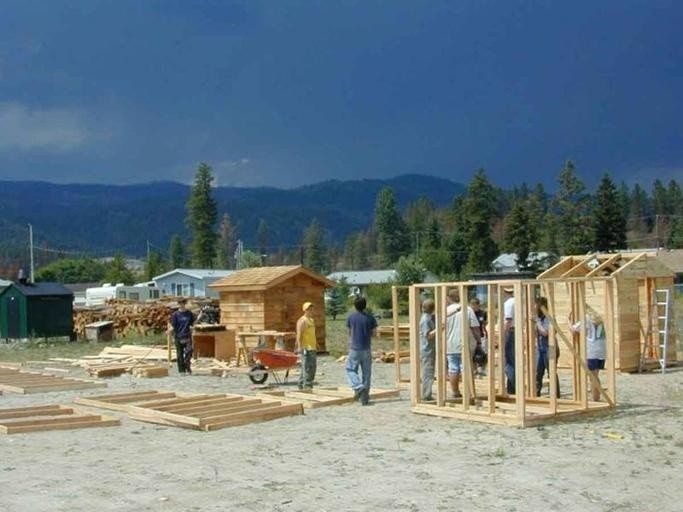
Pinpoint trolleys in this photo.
[248,349,304,385]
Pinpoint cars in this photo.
[492,251,559,272]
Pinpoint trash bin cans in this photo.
[85,320,115,342]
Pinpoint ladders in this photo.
[639,289,670,374]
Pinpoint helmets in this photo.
[473,348,487,366]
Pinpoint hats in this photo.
[302,302,315,310]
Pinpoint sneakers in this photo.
[353,386,366,400]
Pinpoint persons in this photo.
[165,296,196,376]
[344,296,377,405]
[534,295,562,399]
[295,303,318,391]
[502,285,516,395]
[567,301,606,401]
[419,287,487,400]
